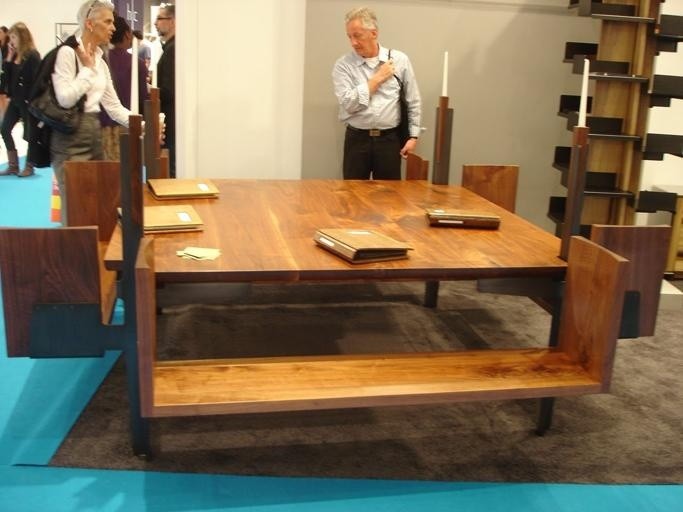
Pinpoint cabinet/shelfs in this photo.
[547,0,681,338]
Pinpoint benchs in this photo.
[0,162,121,358]
[461,164,671,339]
[134,236,629,418]
[158,148,430,181]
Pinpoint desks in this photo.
[104,179,572,457]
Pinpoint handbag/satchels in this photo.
[29,45,83,131]
[387,48,416,150]
[87,0,99,16]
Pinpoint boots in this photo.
[18,150,34,176]
[0,150,18,175]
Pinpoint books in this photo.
[147,176,218,200]
[427,207,500,229]
[315,228,414,265]
[116,205,204,235]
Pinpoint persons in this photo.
[47,0,165,233]
[102,15,148,162]
[331,9,421,179]
[0,23,46,176]
[132,30,149,70]
[1,27,10,117]
[155,6,174,176]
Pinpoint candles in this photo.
[151,45,156,86]
[129,37,139,117]
[578,58,590,127]
[442,51,448,97]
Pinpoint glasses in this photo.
[155,16,171,21]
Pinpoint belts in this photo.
[346,123,399,137]
